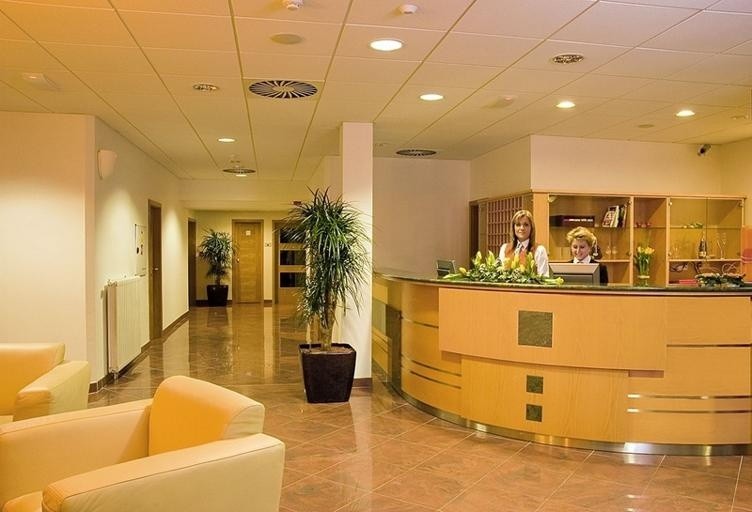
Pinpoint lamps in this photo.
[97,149,119,180]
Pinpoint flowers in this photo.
[690,271,747,287]
[441,249,564,284]
[632,244,657,275]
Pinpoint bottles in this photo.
[699,230,707,259]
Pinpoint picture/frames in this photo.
[601,204,627,227]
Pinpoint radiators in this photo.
[106,276,141,380]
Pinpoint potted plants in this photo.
[199,227,239,308]
[267,185,372,406]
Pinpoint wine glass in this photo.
[713,232,728,259]
[605,243,617,261]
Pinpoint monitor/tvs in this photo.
[548,262,600,285]
[435,259,457,277]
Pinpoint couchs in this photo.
[1,374,287,510]
[0,341,92,418]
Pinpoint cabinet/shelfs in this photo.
[548,194,747,298]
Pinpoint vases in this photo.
[637,274,650,286]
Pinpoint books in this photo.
[602,205,627,228]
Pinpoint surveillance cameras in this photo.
[698,144,712,156]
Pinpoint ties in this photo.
[518,244,522,253]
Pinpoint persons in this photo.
[565,226,608,286]
[498,209,550,278]
